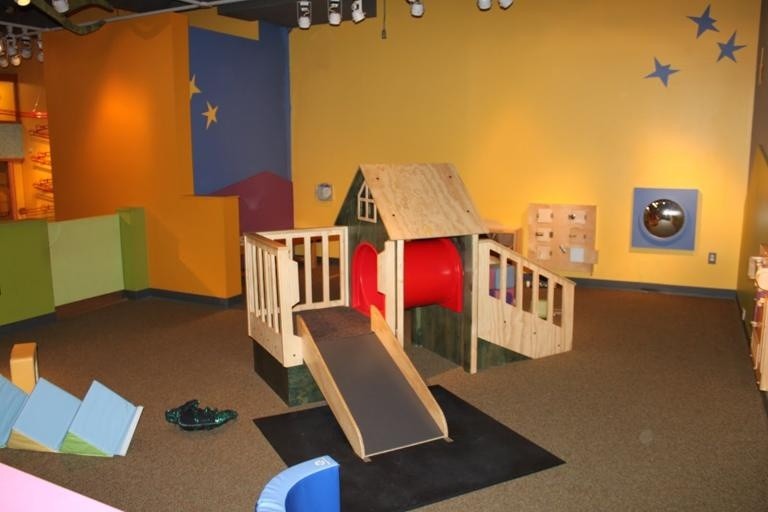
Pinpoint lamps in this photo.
[296,0,513,29]
[0,21,50,68]
[52,0,70,14]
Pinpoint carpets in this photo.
[254,384,566,512]
